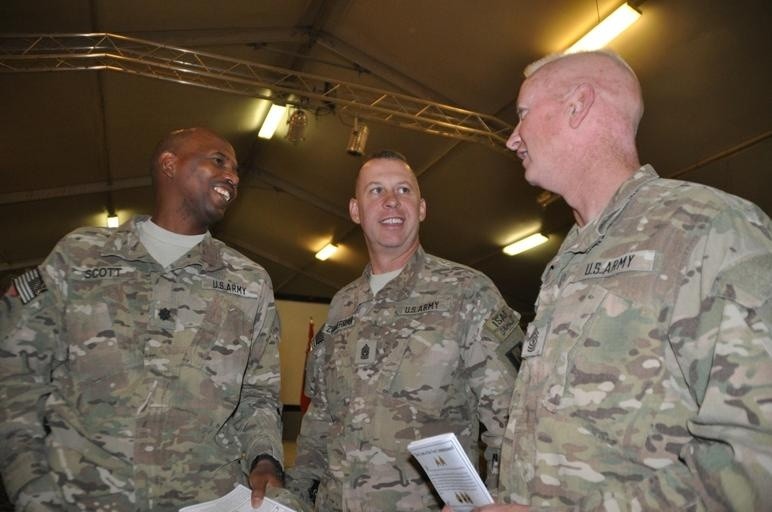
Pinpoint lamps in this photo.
[282,102,369,156]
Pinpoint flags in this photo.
[301,320,315,415]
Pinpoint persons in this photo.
[0,127,285,512]
[439,50,770,512]
[285,149,525,512]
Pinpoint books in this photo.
[406,431,494,512]
[178,484,297,512]
